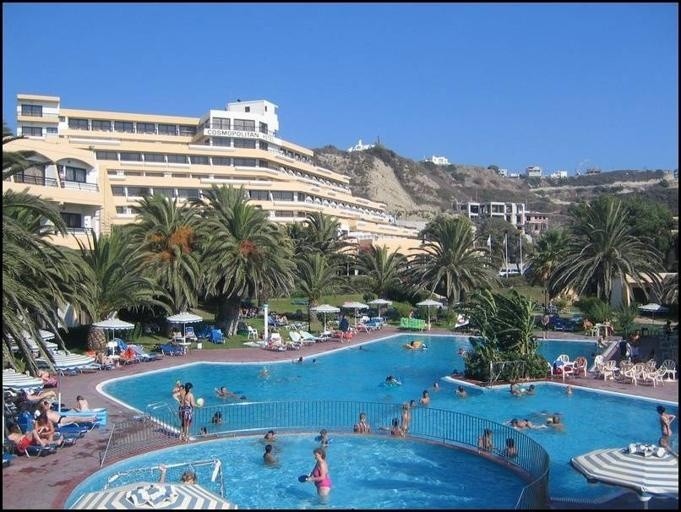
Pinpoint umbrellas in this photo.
[310,303,341,332]
[368,298,393,321]
[342,301,370,328]
[638,302,669,326]
[69,480,241,511]
[571,442,681,509]
[416,299,444,331]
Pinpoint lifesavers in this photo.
[514,385,529,397]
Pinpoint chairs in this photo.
[52,326,198,377]
[247,317,427,351]
[547,322,679,388]
[4,407,107,458]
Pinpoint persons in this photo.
[354,412,370,433]
[541,314,551,339]
[322,429,330,448]
[656,405,677,435]
[629,329,640,344]
[264,430,276,442]
[386,375,401,385]
[263,445,278,464]
[508,379,573,434]
[10,394,96,450]
[95,352,115,367]
[583,317,616,335]
[173,379,234,443]
[297,356,318,363]
[407,340,427,349]
[553,358,588,375]
[391,390,431,437]
[244,308,291,327]
[298,449,332,495]
[455,384,466,396]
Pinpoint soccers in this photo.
[196,398,204,405]
